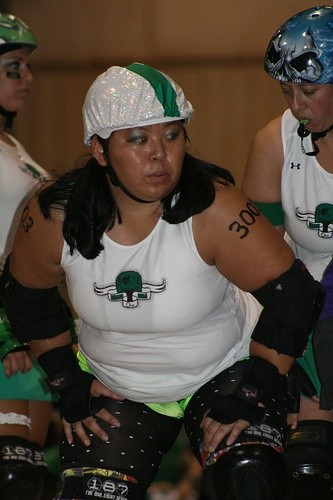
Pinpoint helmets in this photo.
[0,13,38,51]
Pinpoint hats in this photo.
[263,6,332,84]
[83,62,196,144]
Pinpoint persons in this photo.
[1,10,83,499]
[243,5,333,500]
[0,63,325,500]
